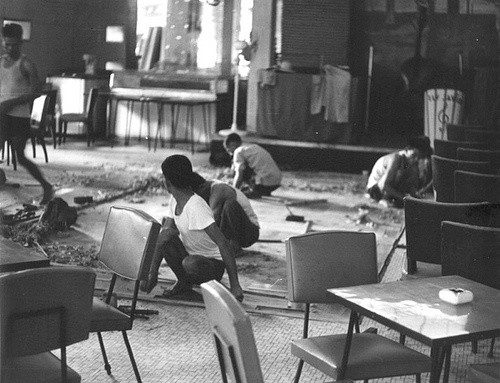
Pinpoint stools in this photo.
[126,79,211,152]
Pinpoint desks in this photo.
[325,274,500,383]
[31,76,109,134]
[0,236,51,273]
[84,88,216,153]
[256,69,367,147]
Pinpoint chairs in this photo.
[0,83,500,383]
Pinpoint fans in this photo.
[220,31,258,137]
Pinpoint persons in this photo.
[0,23,55,205]
[139,154,244,302]
[224,133,282,199]
[190,171,260,257]
[366,138,425,208]
